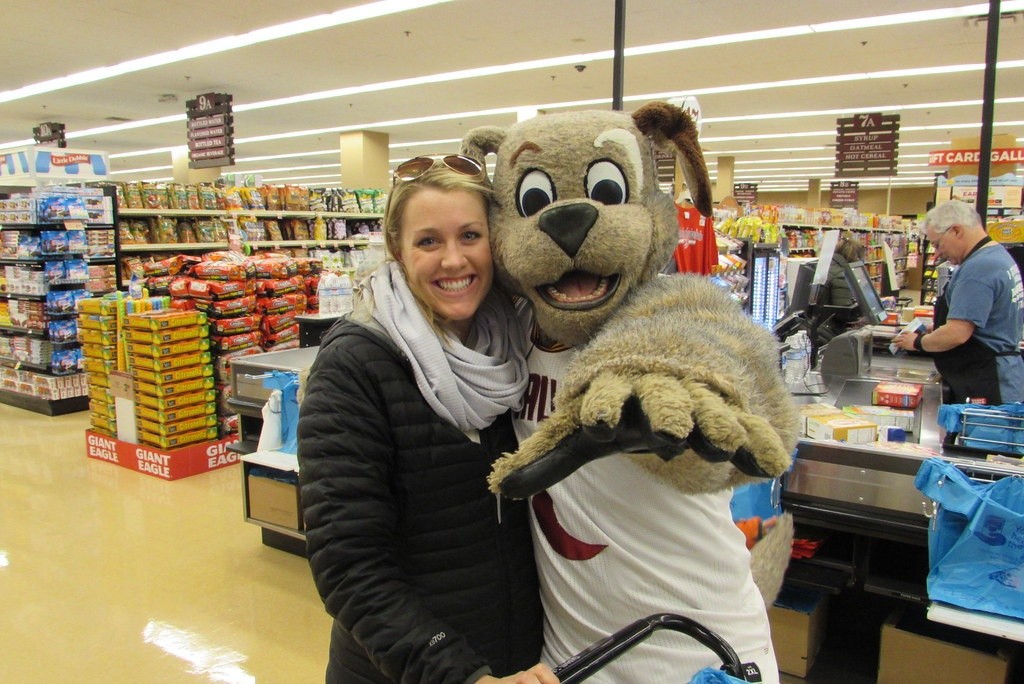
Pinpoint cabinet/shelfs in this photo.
[0,183,387,417]
[770,222,910,303]
[922,227,1024,306]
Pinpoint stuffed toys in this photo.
[461,102,800,684]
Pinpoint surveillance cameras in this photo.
[575,66,586,72]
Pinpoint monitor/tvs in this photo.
[844,262,888,325]
[788,262,818,317]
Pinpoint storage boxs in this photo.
[876,622,1009,684]
[247,476,300,531]
[799,374,922,443]
[0,191,217,450]
[768,591,829,678]
[791,218,1024,306]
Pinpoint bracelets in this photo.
[913,334,925,350]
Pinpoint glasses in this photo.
[932,225,952,249]
[393,153,482,192]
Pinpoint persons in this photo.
[295,154,561,684]
[827,237,865,307]
[891,200,1024,406]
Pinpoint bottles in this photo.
[782,330,811,383]
[319,272,352,313]
